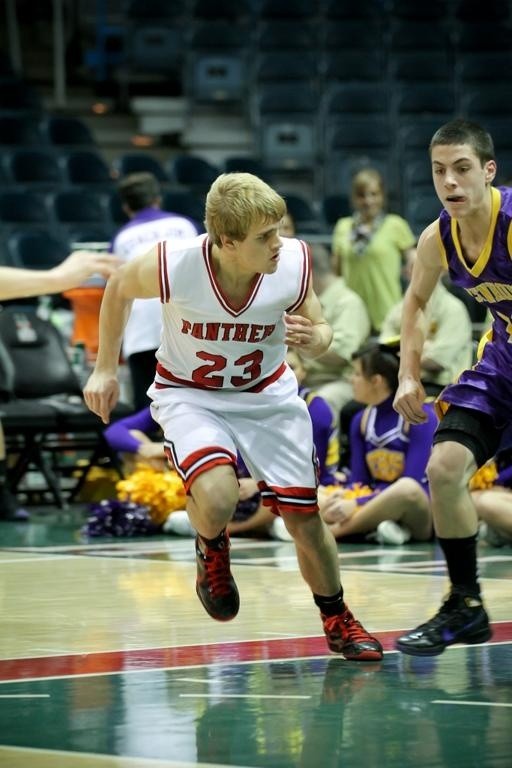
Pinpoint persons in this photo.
[0,248,126,302]
[77,168,387,660]
[59,167,511,551]
[392,120,511,656]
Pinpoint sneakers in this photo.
[377,520,411,546]
[319,608,385,661]
[393,585,492,657]
[478,522,506,548]
[267,516,294,542]
[196,532,240,620]
[169,511,192,537]
